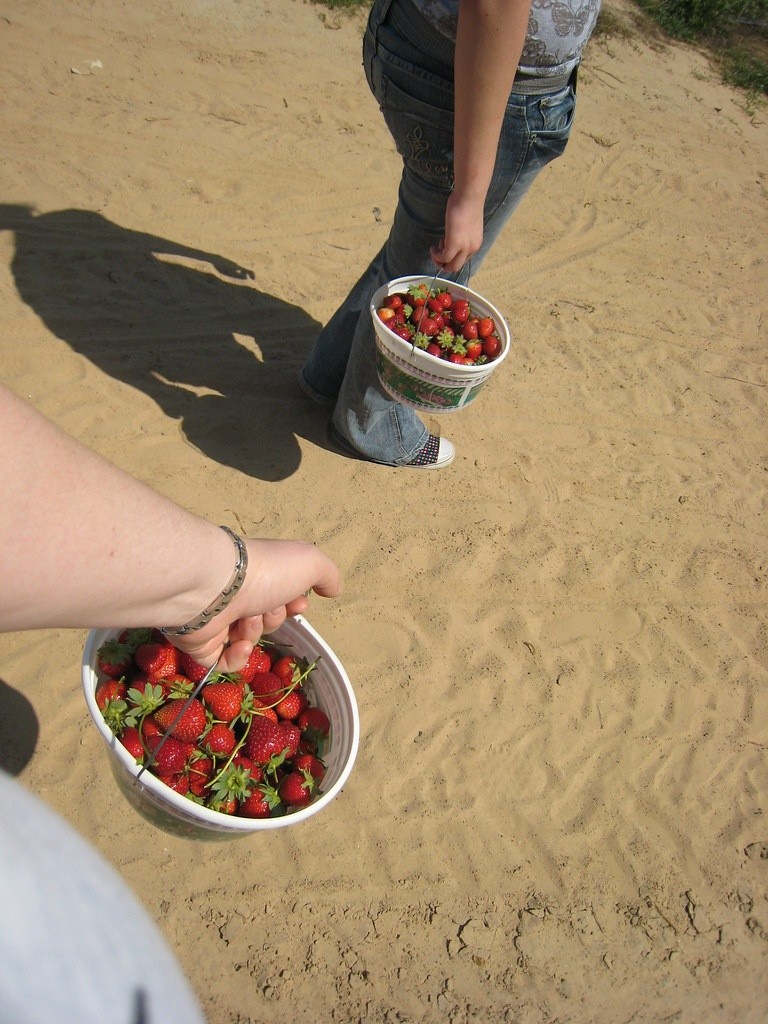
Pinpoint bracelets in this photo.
[156,525,248,637]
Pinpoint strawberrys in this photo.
[378,284,502,366]
[97,628,331,819]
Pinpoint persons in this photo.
[298,0,601,470]
[0,384,345,1024]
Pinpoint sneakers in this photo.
[332,417,457,470]
[295,364,337,408]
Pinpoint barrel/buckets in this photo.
[81,614,360,833]
[370,257,511,413]
